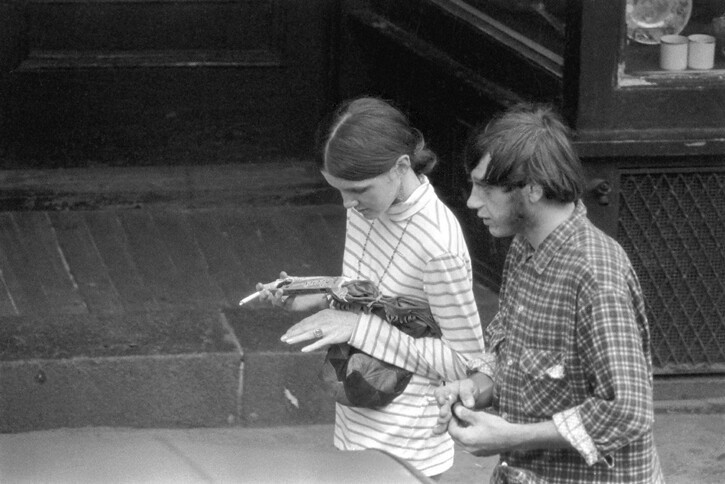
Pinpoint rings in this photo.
[313,328,323,339]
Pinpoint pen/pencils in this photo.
[239,289,265,305]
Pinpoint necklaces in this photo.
[357,215,415,290]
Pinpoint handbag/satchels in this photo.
[324,280,440,409]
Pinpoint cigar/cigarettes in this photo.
[239,289,266,306]
[426,395,449,402]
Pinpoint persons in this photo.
[432,102,665,484]
[254,97,488,484]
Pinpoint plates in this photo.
[625,0,692,44]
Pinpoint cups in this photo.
[659,35,687,71]
[688,34,715,70]
